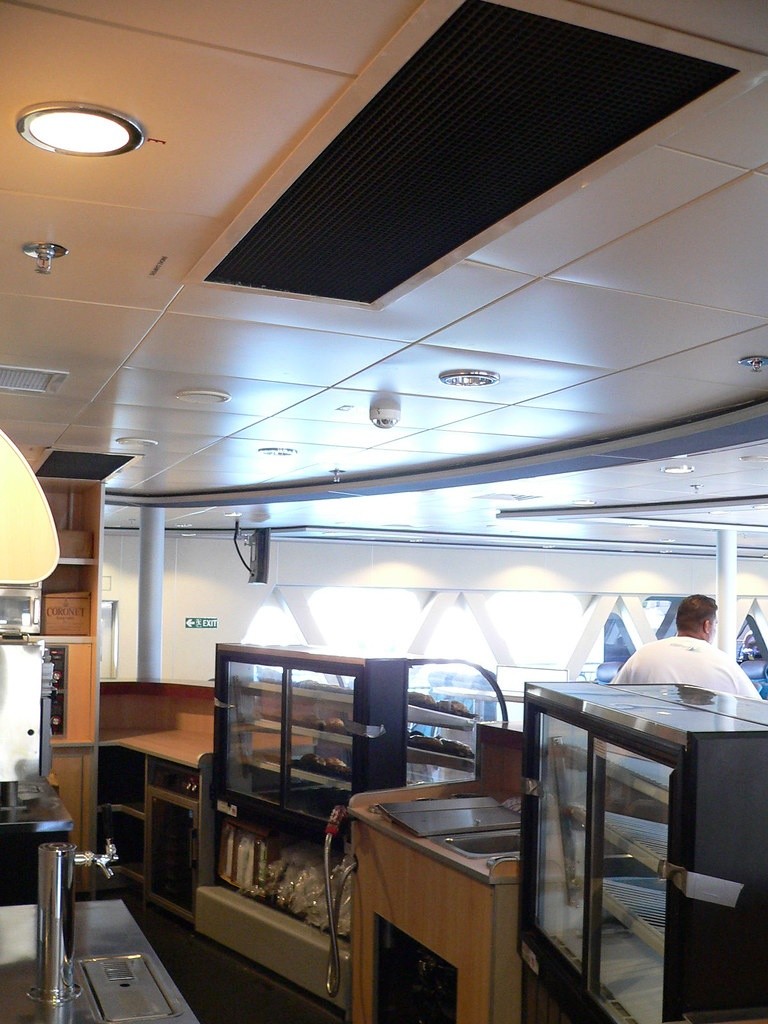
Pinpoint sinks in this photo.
[427,829,520,860]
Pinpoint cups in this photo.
[0,781,18,807]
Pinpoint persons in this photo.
[609,594,762,700]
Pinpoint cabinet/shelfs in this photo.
[32,470,105,896]
[98,726,214,926]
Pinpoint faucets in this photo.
[75,838,118,878]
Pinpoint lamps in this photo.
[246,527,270,585]
